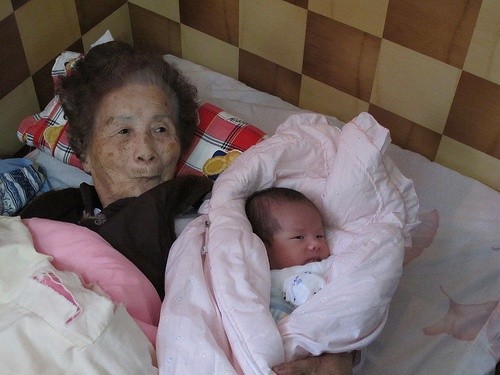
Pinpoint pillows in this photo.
[17,86,270,235]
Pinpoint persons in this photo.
[244,187,329,375]
[14,40,366,375]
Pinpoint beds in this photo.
[0,55,500,375]
